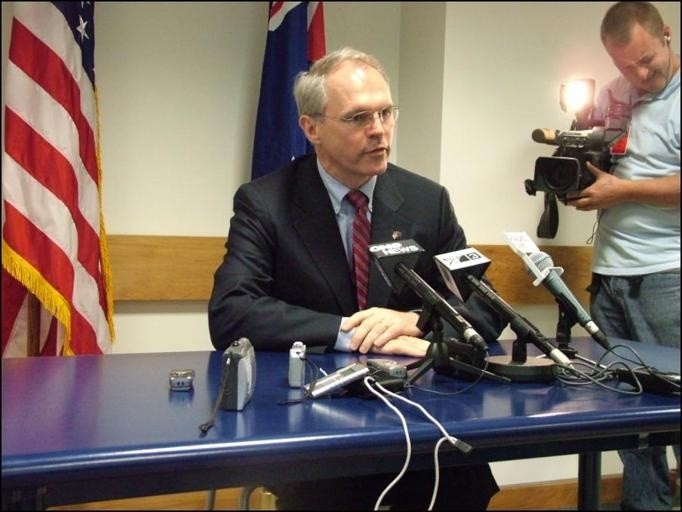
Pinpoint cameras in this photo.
[217,337,258,411]
[286,340,307,387]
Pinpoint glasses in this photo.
[341,105,400,128]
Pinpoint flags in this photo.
[251,1,327,182]
[1,1,115,359]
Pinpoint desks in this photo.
[1,336,681,510]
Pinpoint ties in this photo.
[346,190,371,311]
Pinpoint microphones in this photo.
[529,250,612,350]
[368,236,489,355]
[436,245,577,375]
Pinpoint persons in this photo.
[570,1,681,511]
[208,47,509,512]
